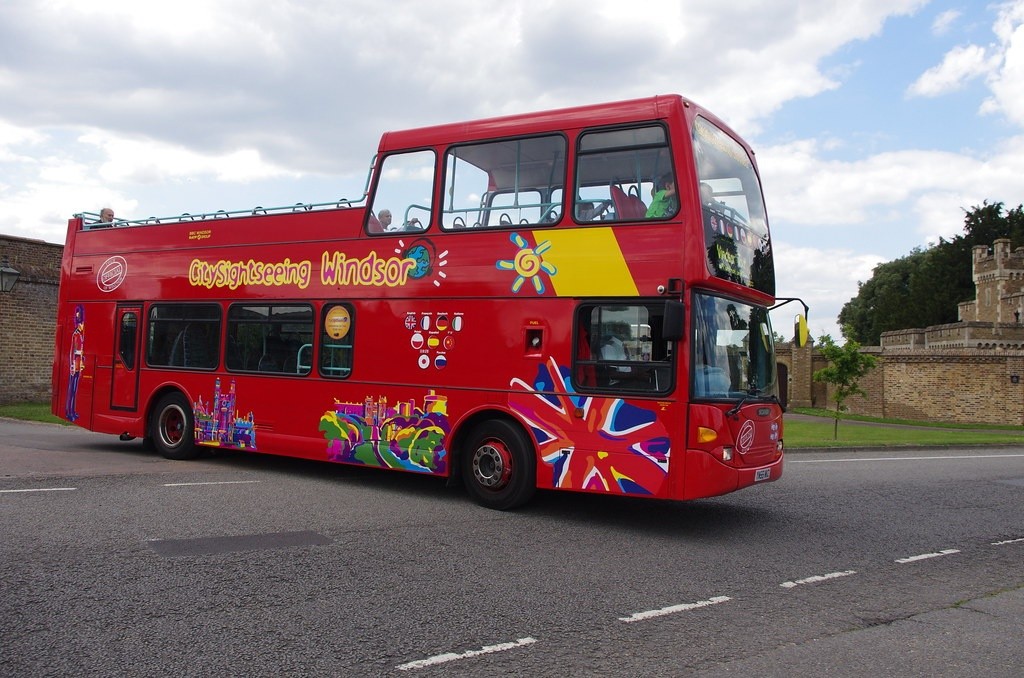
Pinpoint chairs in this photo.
[168,322,341,377]
[115,199,384,233]
[390,176,647,239]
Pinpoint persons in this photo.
[552,201,614,223]
[378,209,418,232]
[89,208,114,229]
[233,337,310,375]
[643,171,714,219]
[599,319,651,386]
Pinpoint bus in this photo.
[49,92,809,511]
